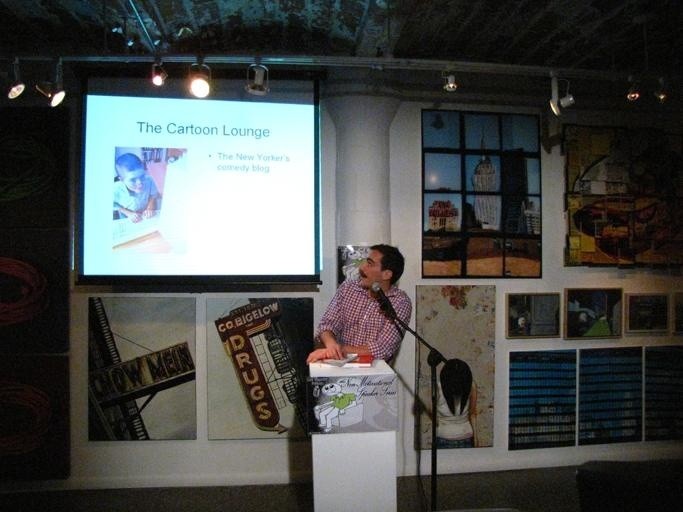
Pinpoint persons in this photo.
[304,244,414,370]
[430,357,482,448]
[113,152,160,224]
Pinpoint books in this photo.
[321,354,357,366]
[343,353,372,366]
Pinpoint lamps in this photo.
[0,53,683,114]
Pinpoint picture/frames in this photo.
[503,287,683,340]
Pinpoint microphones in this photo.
[371,282,397,318]
